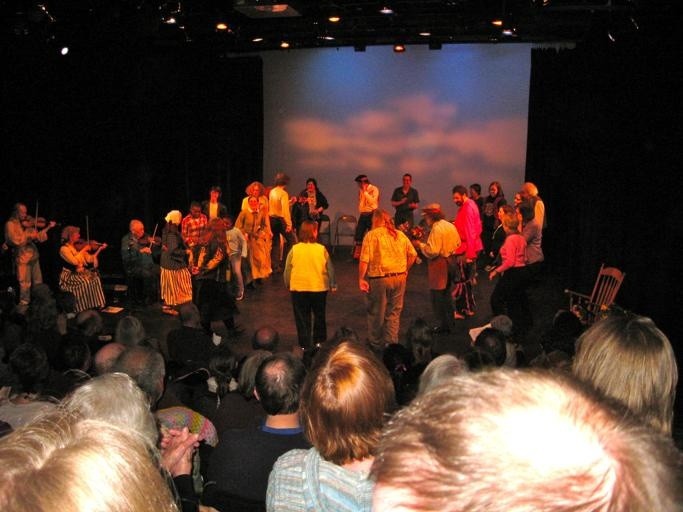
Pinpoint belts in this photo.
[368,270,408,280]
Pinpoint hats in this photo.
[420,202,440,216]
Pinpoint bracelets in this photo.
[494,269,498,273]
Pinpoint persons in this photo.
[94,342,185,413]
[55,290,78,336]
[4,202,57,307]
[469,183,484,262]
[470,315,518,369]
[358,208,418,362]
[452,185,484,319]
[484,204,516,278]
[52,332,92,383]
[515,202,546,331]
[180,200,208,266]
[292,191,310,242]
[514,190,530,207]
[114,314,160,353]
[190,348,240,422]
[230,324,305,384]
[268,177,295,275]
[283,219,338,356]
[58,225,107,312]
[234,195,273,289]
[265,172,297,271]
[241,181,274,285]
[366,366,683,512]
[120,219,161,306]
[200,185,228,224]
[264,338,395,511]
[187,217,246,341]
[297,177,329,237]
[488,212,529,334]
[58,371,202,512]
[199,351,314,512]
[412,203,462,336]
[222,214,248,301]
[0,339,58,432]
[521,182,546,231]
[112,345,204,494]
[159,210,193,317]
[23,282,58,343]
[166,302,231,402]
[353,174,380,260]
[0,402,181,511]
[390,173,421,242]
[210,348,274,433]
[482,181,507,258]
[60,310,109,365]
[570,312,679,439]
[416,353,470,396]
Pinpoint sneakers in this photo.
[236,288,244,301]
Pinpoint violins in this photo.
[74,239,113,252]
[22,214,62,229]
[138,236,163,246]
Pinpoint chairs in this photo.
[563,264,626,328]
[317,214,332,244]
[335,214,356,257]
